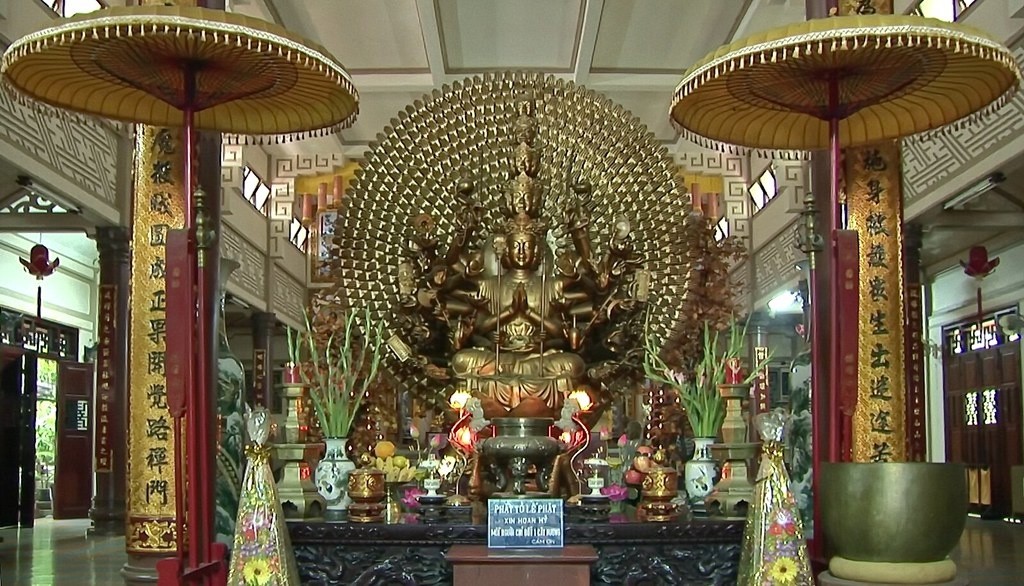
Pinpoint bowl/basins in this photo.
[817,460,970,583]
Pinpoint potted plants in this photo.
[288,306,386,508]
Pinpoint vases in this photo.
[213,255,248,557]
[687,436,721,507]
[786,258,813,538]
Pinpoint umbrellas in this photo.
[667,16,1022,557]
[1,6,360,568]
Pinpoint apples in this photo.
[626,447,656,484]
[394,456,407,468]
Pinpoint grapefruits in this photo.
[374,441,396,457]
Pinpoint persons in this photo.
[452,91,600,407]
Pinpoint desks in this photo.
[445,544,598,586]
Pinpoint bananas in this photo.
[376,456,417,481]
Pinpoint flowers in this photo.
[636,315,775,435]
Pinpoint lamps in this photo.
[944,175,1005,212]
[228,294,250,311]
[14,174,82,215]
